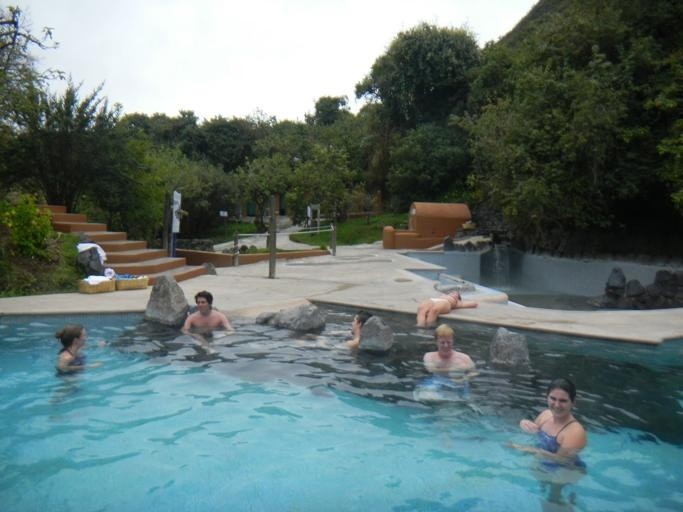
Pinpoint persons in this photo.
[413,326,482,417]
[54,324,107,373]
[345,312,372,350]
[181,291,232,340]
[415,291,478,332]
[512,378,587,468]
